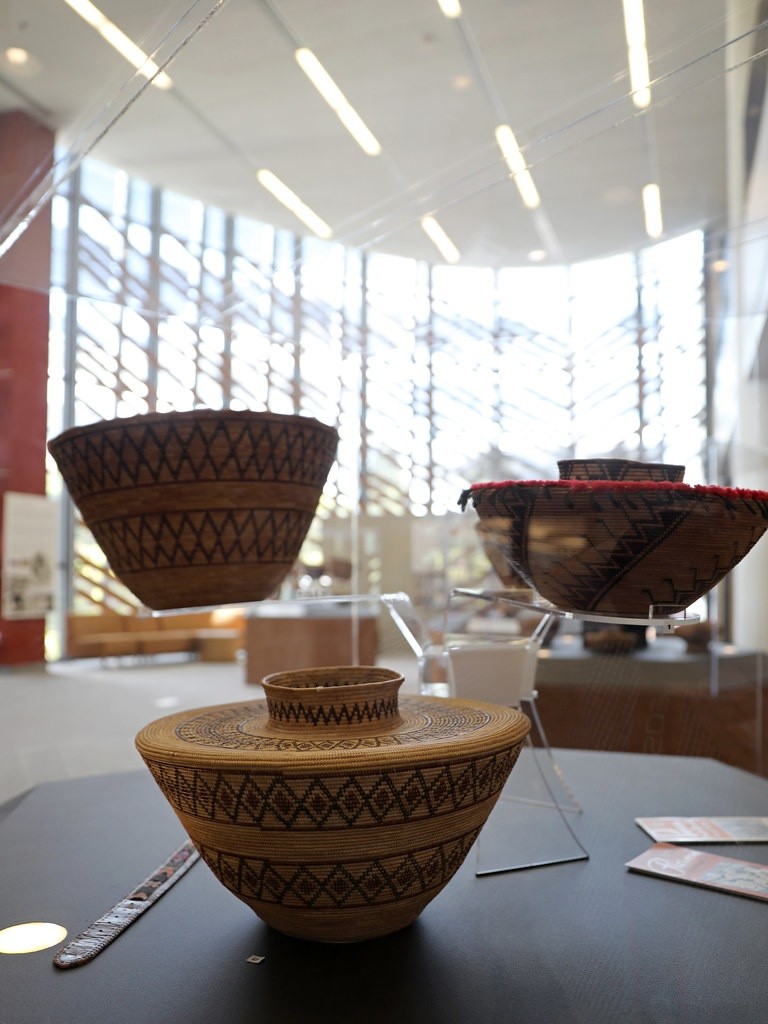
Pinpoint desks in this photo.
[0,748,767,1024]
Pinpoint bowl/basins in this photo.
[674,622,724,653]
[584,632,639,654]
